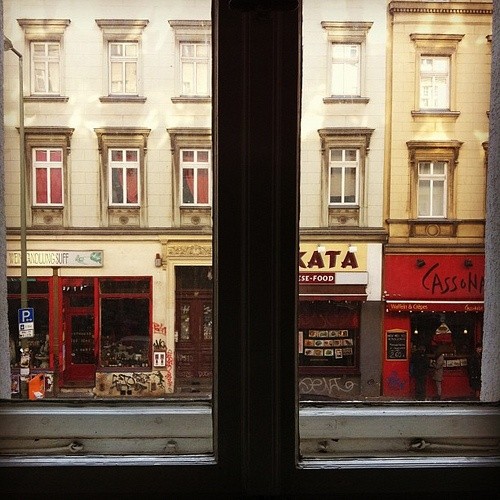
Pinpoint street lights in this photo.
[3,34,33,399]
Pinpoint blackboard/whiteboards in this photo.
[385,328,409,362]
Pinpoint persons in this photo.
[466,342,483,400]
[410,343,429,401]
[428,351,445,401]
